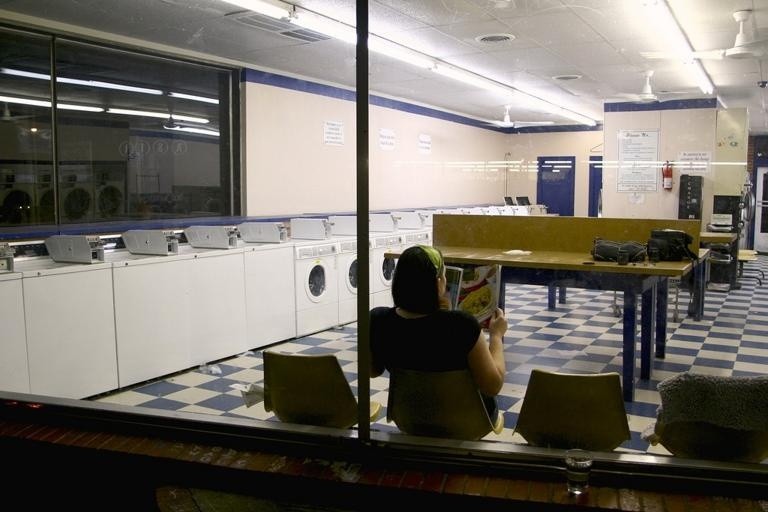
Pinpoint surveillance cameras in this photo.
[758,82,768,89]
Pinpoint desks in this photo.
[388,204,707,398]
[699,231,738,293]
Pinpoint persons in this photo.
[369,245,511,430]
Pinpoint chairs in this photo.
[254,351,379,430]
[513,363,633,454]
[392,368,504,441]
[653,373,767,465]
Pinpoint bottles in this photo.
[618,250,629,264]
[649,247,660,261]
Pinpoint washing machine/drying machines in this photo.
[0,162,126,228]
[289,196,560,340]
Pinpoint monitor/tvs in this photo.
[712,195,742,228]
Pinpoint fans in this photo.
[659,7,767,63]
[602,69,697,105]
[474,102,553,131]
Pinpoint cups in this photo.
[565,448,594,495]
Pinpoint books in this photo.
[443,261,507,330]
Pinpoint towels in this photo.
[643,372,768,445]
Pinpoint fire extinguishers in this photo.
[662,160,675,189]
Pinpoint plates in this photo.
[461,284,497,322]
[460,266,486,288]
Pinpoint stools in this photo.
[737,247,766,280]
[737,253,761,286]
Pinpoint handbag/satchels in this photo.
[650,227,693,263]
[592,239,646,262]
